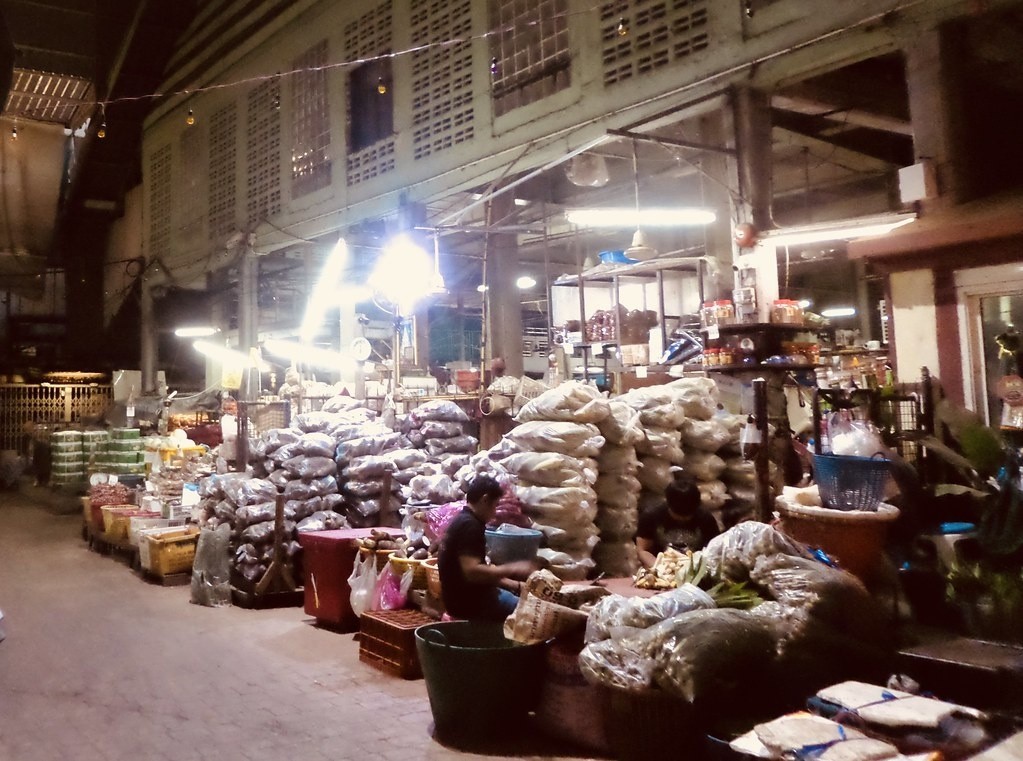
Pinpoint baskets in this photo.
[81,496,92,522]
[130,516,185,544]
[137,525,191,569]
[146,530,200,574]
[812,451,892,512]
[359,545,401,570]
[162,502,192,520]
[91,501,126,532]
[514,375,551,406]
[421,558,444,598]
[101,505,139,536]
[388,549,430,588]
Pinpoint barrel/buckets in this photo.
[414,621,556,743]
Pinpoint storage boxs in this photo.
[614,372,677,395]
[138,523,192,570]
[146,528,202,575]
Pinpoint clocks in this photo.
[350,337,372,362]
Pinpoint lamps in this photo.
[10,114,18,142]
[186,92,195,125]
[584,238,595,271]
[623,140,659,262]
[377,59,385,93]
[618,2,626,35]
[490,34,497,74]
[97,103,106,138]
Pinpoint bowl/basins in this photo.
[485,528,542,564]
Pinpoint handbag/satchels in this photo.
[347,550,380,617]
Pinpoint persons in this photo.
[637,480,720,570]
[438,476,533,620]
[221,398,255,443]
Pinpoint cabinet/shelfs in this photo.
[553,257,705,391]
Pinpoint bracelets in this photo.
[519,582,520,590]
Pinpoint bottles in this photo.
[699,300,805,365]
